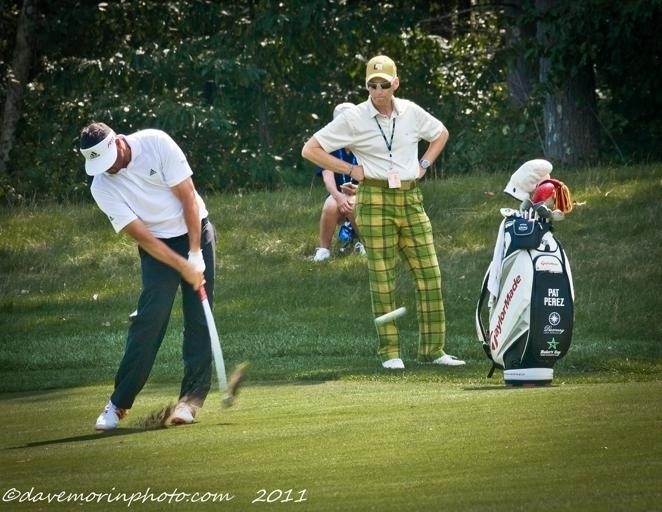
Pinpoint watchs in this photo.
[418,158,433,173]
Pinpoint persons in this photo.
[312,101,368,264]
[79,121,219,432]
[298,53,467,371]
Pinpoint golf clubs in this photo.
[500,159,572,223]
[200,279,249,409]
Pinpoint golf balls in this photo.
[375,307,408,328]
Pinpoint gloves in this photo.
[187,248,206,272]
[502,158,554,203]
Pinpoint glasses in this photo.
[366,81,392,91]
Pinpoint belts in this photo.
[363,177,417,192]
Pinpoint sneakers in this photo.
[423,353,466,366]
[311,247,332,263]
[382,357,406,370]
[92,399,130,432]
[169,403,197,425]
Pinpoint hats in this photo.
[80,130,118,175]
[363,55,398,90]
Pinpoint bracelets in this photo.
[346,164,355,177]
